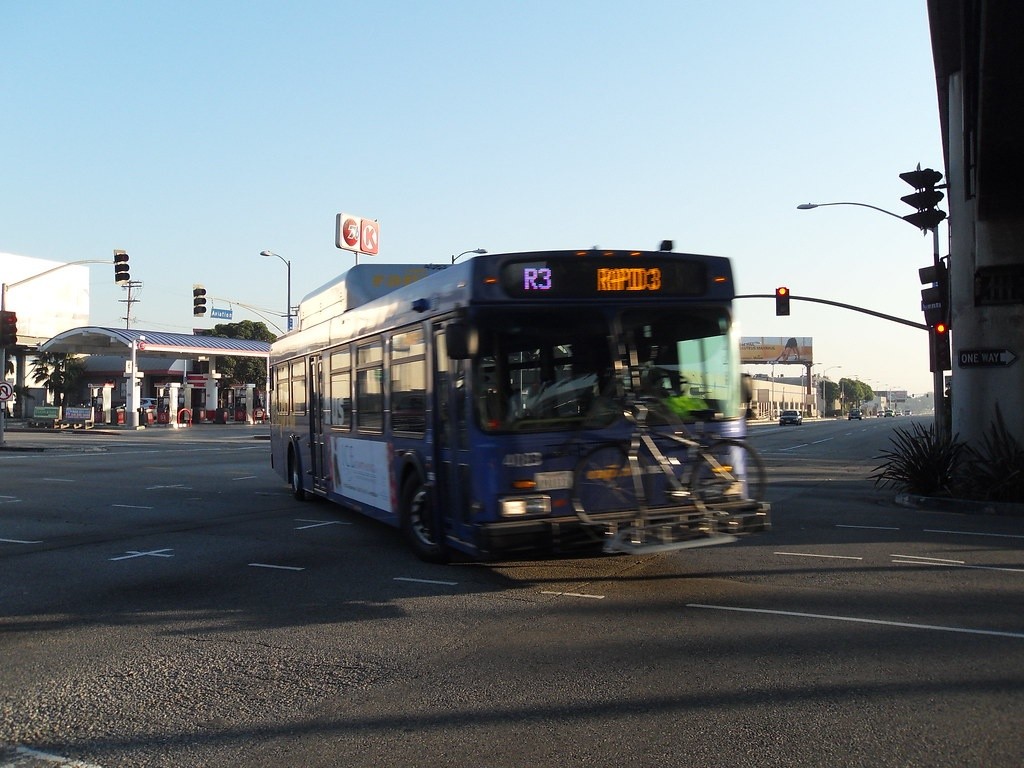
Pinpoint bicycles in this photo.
[569,403,767,531]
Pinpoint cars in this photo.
[116,398,157,420]
[778,407,912,426]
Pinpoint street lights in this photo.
[450,247,486,268]
[260,251,293,331]
[796,199,948,449]
[800,360,902,418]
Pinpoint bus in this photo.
[264,242,751,565]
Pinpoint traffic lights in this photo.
[917,259,949,322]
[775,286,788,315]
[192,284,207,316]
[930,321,950,369]
[114,250,131,285]
